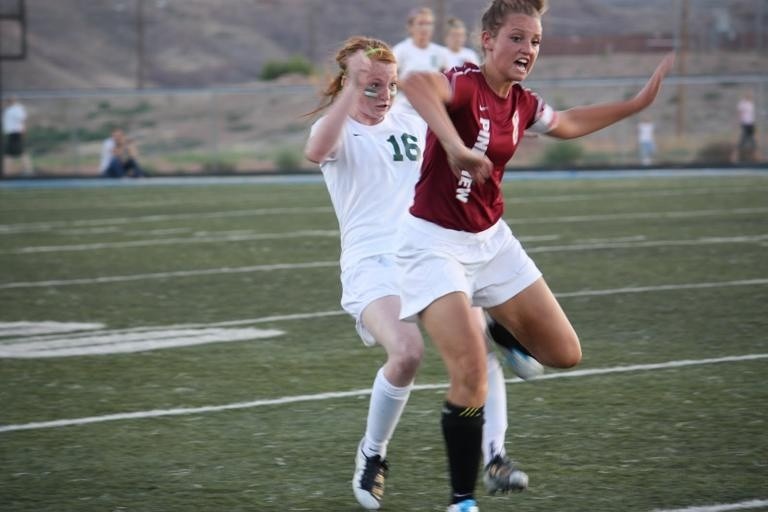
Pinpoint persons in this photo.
[98,128,150,178]
[305,37,529,509]
[739,86,760,166]
[637,114,656,166]
[388,8,450,76]
[398,0,672,512]
[2,93,33,177]
[443,16,482,70]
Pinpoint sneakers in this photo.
[352,435,389,512]
[484,318,545,380]
[446,499,479,512]
[484,455,529,498]
